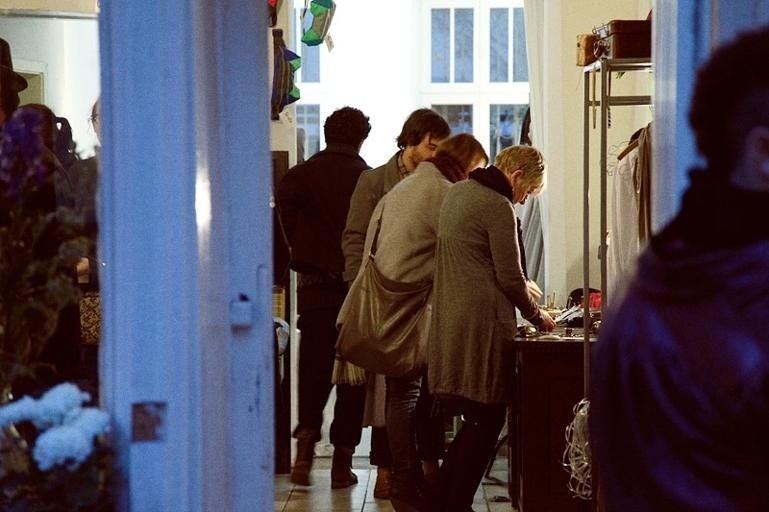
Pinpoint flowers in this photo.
[1,380,130,512]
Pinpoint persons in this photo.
[423,144,559,510]
[338,132,489,504]
[339,107,452,500]
[272,107,380,490]
[587,23,766,509]
[0,36,89,337]
[16,104,87,378]
[71,93,103,262]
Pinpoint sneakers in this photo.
[374,460,474,511]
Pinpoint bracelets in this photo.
[520,302,541,319]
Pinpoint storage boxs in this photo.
[595,20,651,58]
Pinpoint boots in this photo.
[288,428,316,492]
[331,446,358,489]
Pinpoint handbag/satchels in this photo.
[333,259,434,380]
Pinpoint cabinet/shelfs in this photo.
[507,332,599,510]
